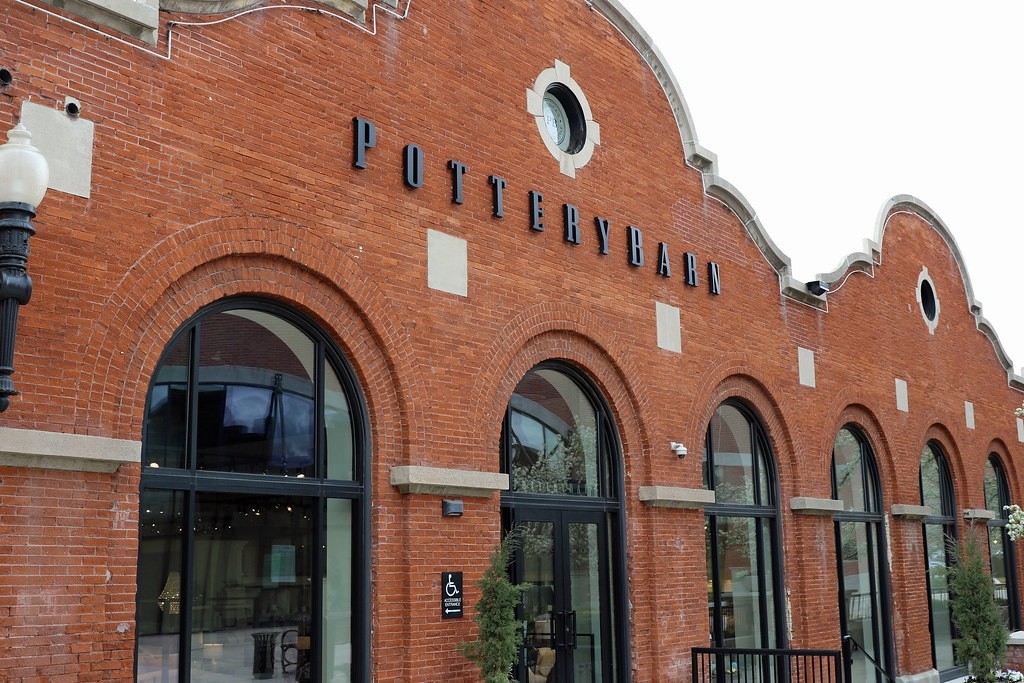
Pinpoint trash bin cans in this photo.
[252,630,279,680]
